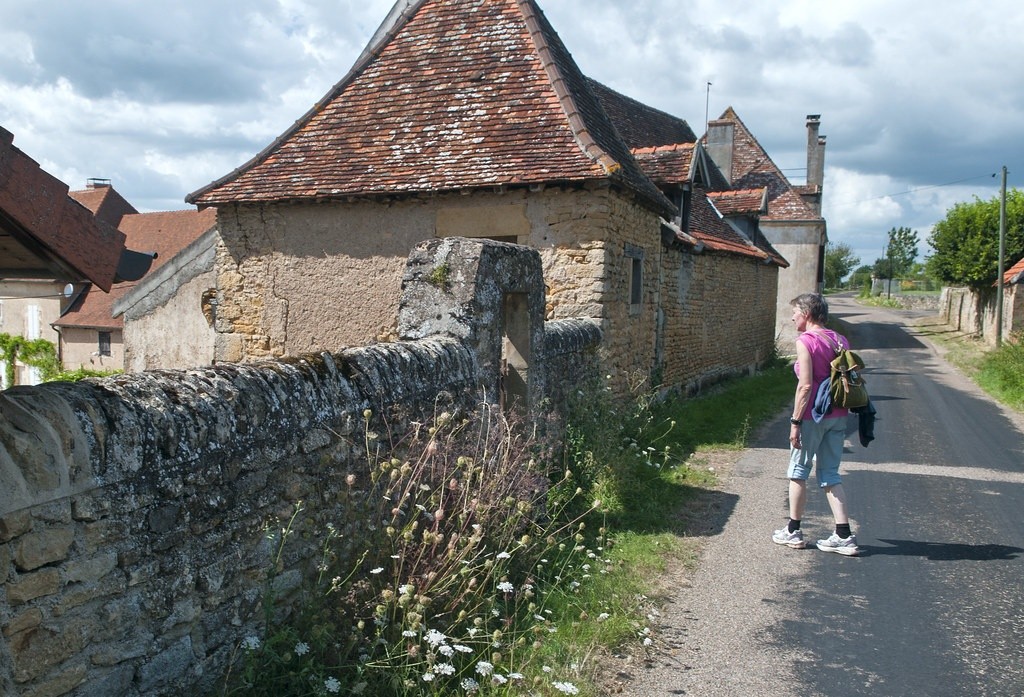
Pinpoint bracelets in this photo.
[790,417,801,425]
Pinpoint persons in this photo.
[772,293,861,556]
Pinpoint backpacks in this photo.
[810,330,869,409]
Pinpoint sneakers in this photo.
[772,526,806,549]
[816,531,860,555]
